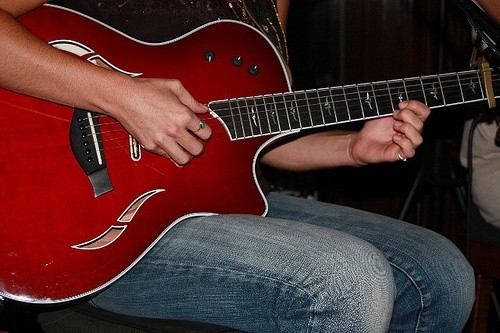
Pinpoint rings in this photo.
[398,153,407,162]
[194,121,205,133]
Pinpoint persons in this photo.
[0,0,476,332]
[458,1,500,230]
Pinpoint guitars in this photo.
[1,3,496,307]
